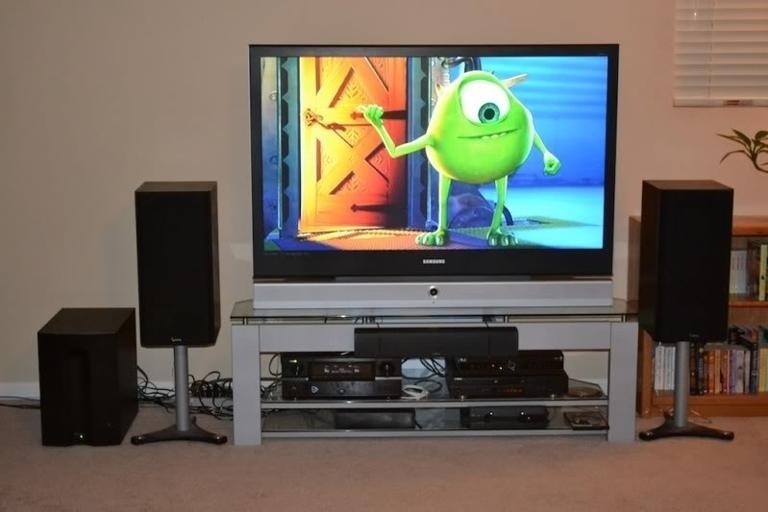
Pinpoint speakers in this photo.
[37,308,139,447]
[135,181,220,347]
[638,180,734,343]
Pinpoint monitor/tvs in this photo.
[248,44,619,317]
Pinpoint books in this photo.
[755,239,768,302]
[728,249,747,295]
[652,321,767,396]
[562,407,609,433]
[744,239,760,302]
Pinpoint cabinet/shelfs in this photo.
[624,213,768,419]
[230,299,641,445]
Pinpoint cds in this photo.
[567,386,598,397]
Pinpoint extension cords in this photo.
[189,394,233,408]
